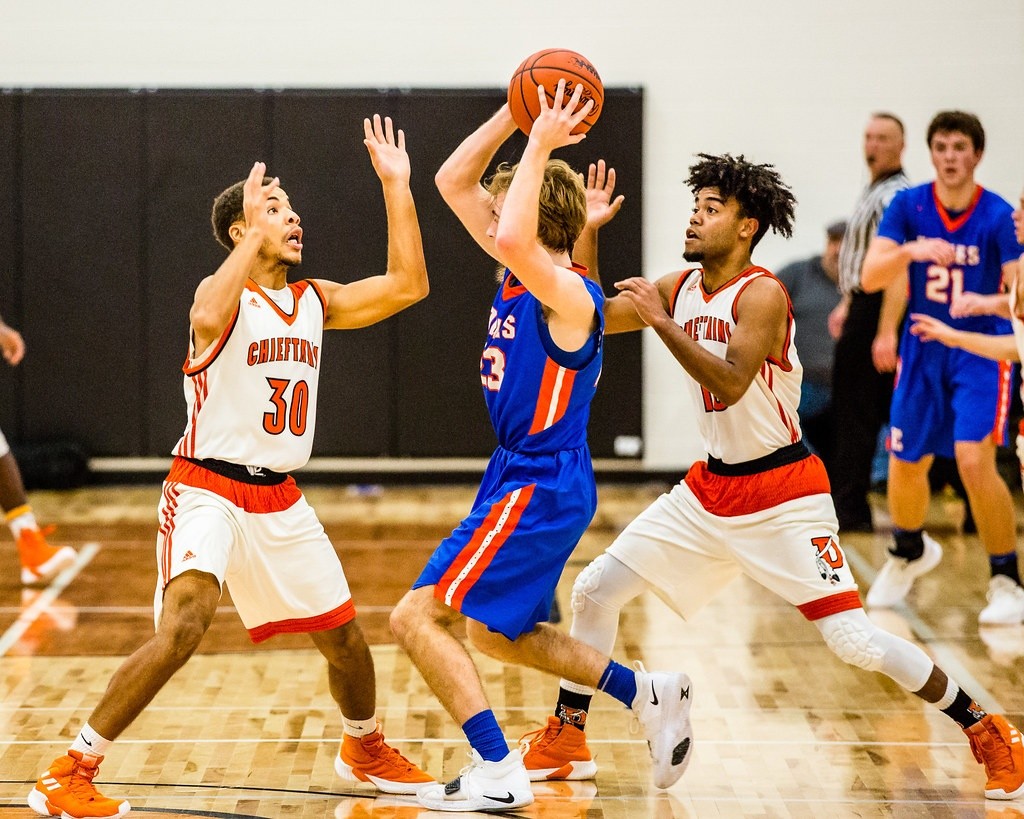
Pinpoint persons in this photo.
[25,114,447,819]
[773,222,849,416]
[858,108,1024,626]
[911,187,1024,498]
[828,111,916,533]
[521,152,1024,802]
[0,318,80,585]
[387,77,692,814]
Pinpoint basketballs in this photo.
[507,50,602,136]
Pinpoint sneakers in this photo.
[416,748,534,811]
[333,719,438,794]
[27,749,131,819]
[627,660,694,788]
[977,574,1024,624]
[15,526,78,586]
[519,715,598,781]
[963,714,1024,799]
[865,531,943,606]
